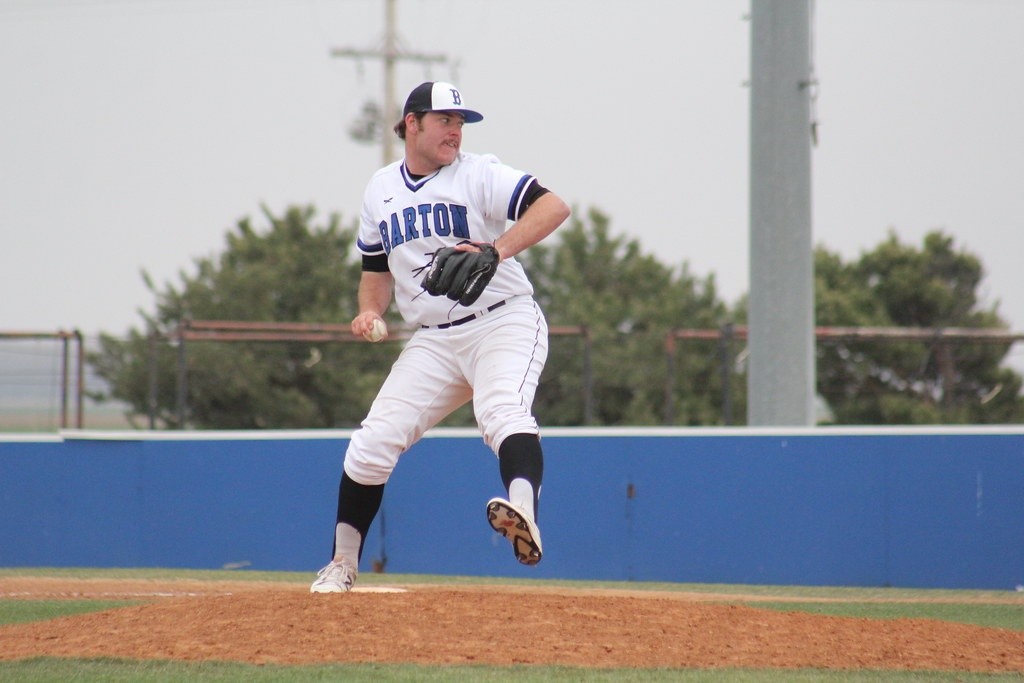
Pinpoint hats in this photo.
[404,81,484,124]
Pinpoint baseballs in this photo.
[362,319,387,343]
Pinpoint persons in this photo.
[310,82,571,594]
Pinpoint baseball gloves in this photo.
[419,238,501,308]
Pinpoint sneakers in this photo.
[310,555,358,593]
[486,497,543,565]
